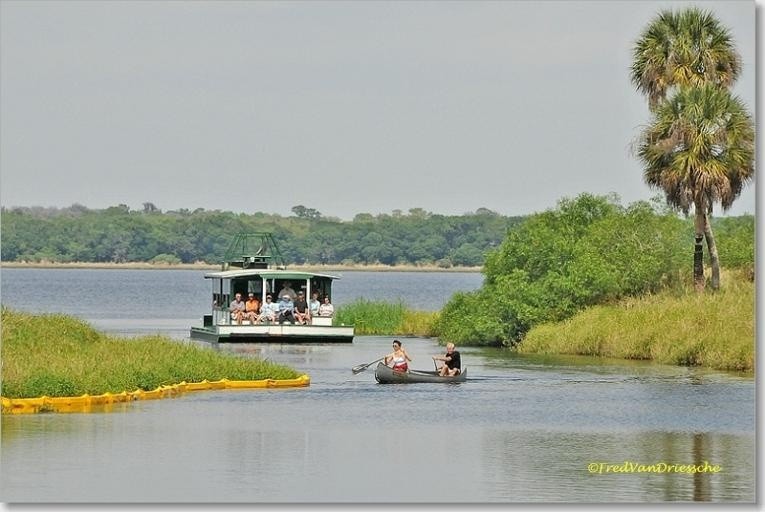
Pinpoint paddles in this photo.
[352,354,393,374]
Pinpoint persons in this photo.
[385,340,410,372]
[432,342,461,375]
[230,281,334,325]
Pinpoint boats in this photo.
[190,233,355,344]
[374,361,468,385]
